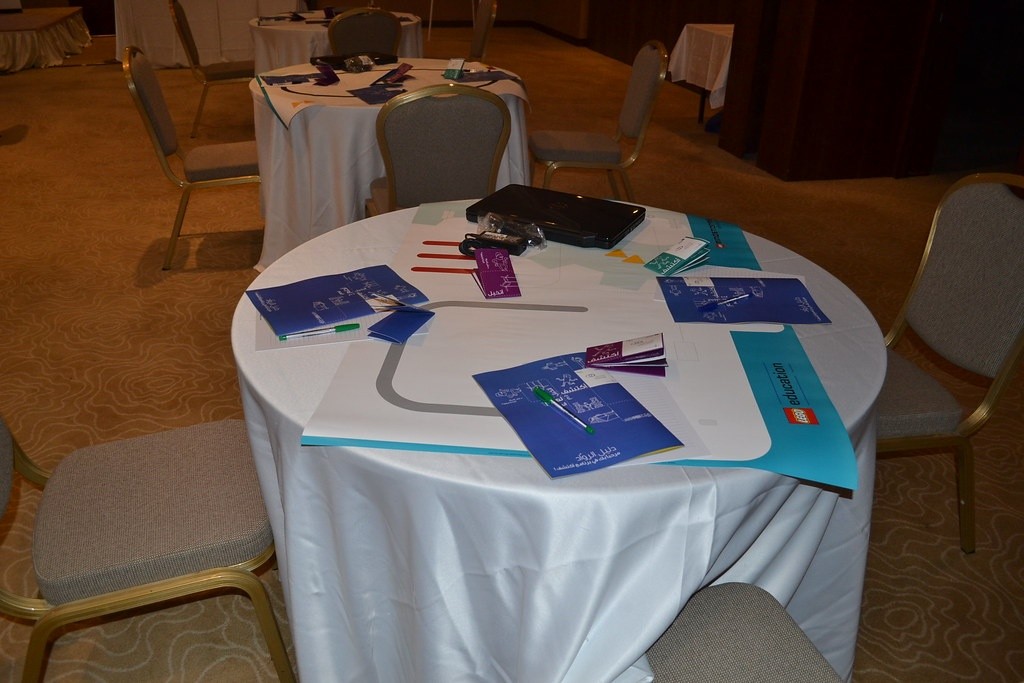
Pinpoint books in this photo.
[656,276,832,324]
[346,85,393,105]
[472,351,685,479]
[244,264,430,336]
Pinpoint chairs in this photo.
[0,415,298,683]
[876,172,1024,554]
[328,8,403,56]
[365,83,511,218]
[123,45,261,271]
[529,39,667,204]
[650,582,846,682]
[467,0,497,62]
[168,0,256,139]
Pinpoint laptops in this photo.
[466,184,647,249]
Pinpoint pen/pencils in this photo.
[280,322,361,341]
[269,81,302,87]
[370,83,403,87]
[531,386,594,434]
[463,68,490,73]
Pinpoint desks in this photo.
[114,0,308,68]
[248,58,533,274]
[248,9,424,79]
[231,196,887,683]
[667,23,734,123]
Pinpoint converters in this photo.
[476,231,527,256]
